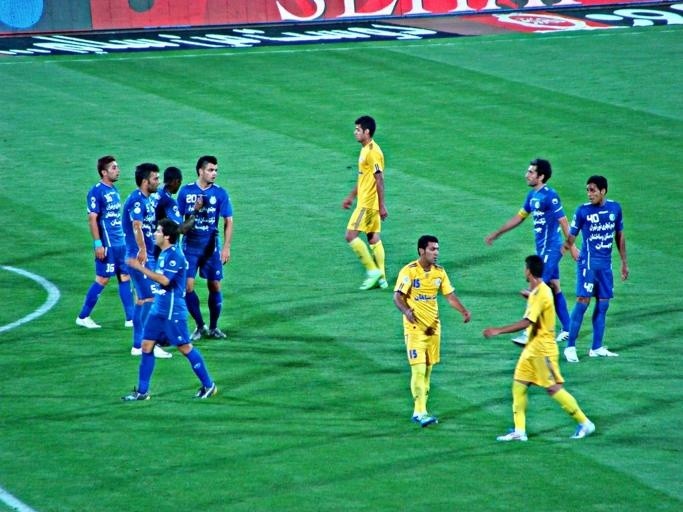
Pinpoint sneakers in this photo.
[207,326,228,341]
[588,346,620,358]
[555,330,571,344]
[563,346,580,363]
[511,331,528,346]
[190,381,219,402]
[119,387,152,404]
[494,430,530,444]
[189,323,208,342]
[357,268,389,292]
[74,312,103,330]
[123,316,181,359]
[569,420,596,440]
[410,412,439,429]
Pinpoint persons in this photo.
[75,155,234,403]
[340,115,389,292]
[481,256,596,442]
[559,175,630,364]
[392,235,471,427]
[484,159,581,347]
